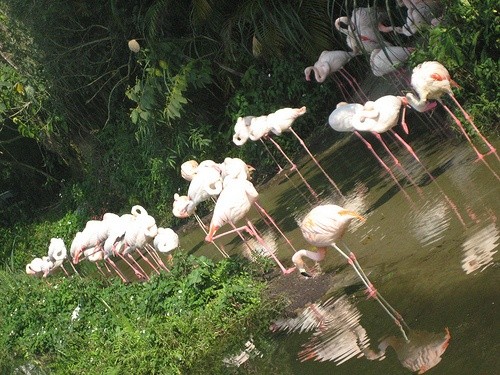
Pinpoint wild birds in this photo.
[370,46,415,93]
[407,60,496,158]
[232,105,306,171]
[171,156,296,275]
[26,237,78,278]
[69,205,180,284]
[394,0,443,37]
[291,204,378,300]
[362,94,420,164]
[304,49,368,100]
[335,7,390,53]
[328,101,399,171]
